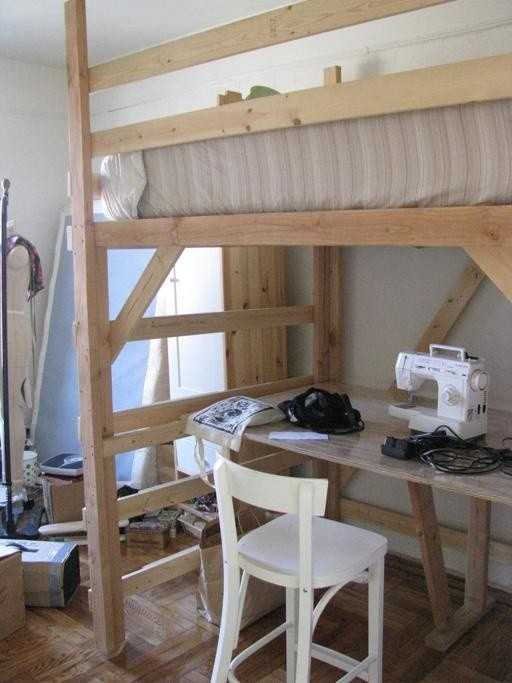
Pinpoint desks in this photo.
[185,382,511,652]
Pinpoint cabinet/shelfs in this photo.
[156,247,288,476]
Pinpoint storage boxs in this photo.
[42,474,85,523]
[0,539,81,609]
[0,547,26,640]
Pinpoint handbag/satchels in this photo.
[183,388,290,492]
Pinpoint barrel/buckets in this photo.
[24,427,36,452]
[23,450,38,486]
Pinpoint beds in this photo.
[65,0,510,657]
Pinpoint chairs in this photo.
[210,452,388,683]
